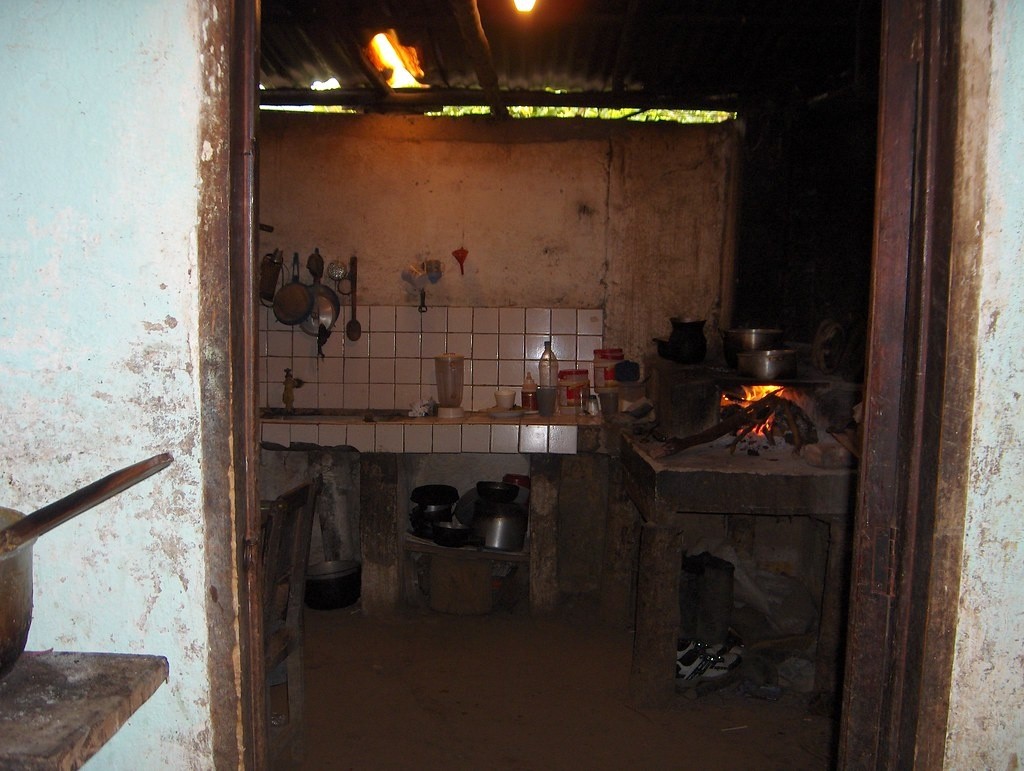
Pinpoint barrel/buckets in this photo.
[501,472,531,504]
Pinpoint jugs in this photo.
[433,353,466,407]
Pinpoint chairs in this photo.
[260,472,326,771]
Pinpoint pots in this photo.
[652,337,669,357]
[717,326,784,367]
[410,505,453,539]
[305,559,361,610]
[273,251,341,337]
[409,485,458,505]
[472,501,527,553]
[750,344,798,381]
[431,521,470,548]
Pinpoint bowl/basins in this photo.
[477,481,520,503]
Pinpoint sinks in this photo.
[265,412,402,423]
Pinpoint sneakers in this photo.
[676,639,745,680]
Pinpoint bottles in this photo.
[593,348,625,394]
[558,369,590,413]
[539,340,559,388]
[589,395,599,416]
[520,373,539,411]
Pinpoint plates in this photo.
[486,410,524,418]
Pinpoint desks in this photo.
[0,652,171,771]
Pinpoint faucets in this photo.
[282,368,303,416]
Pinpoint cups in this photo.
[494,391,516,411]
[599,393,619,413]
[401,259,428,290]
[668,316,707,364]
[537,386,558,416]
